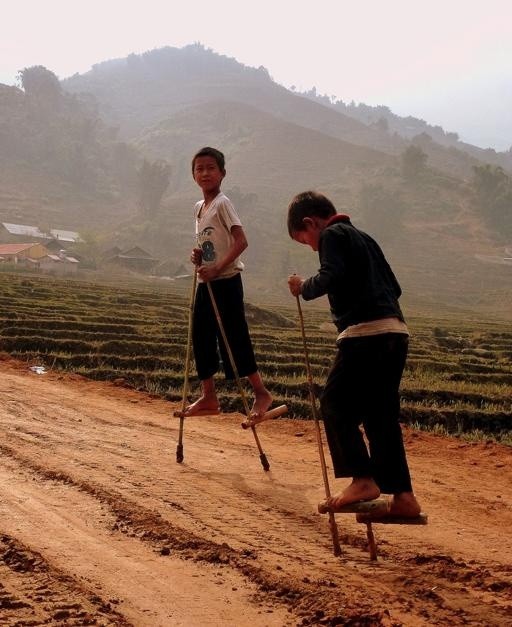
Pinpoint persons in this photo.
[287,189,423,523]
[181,145,273,419]
